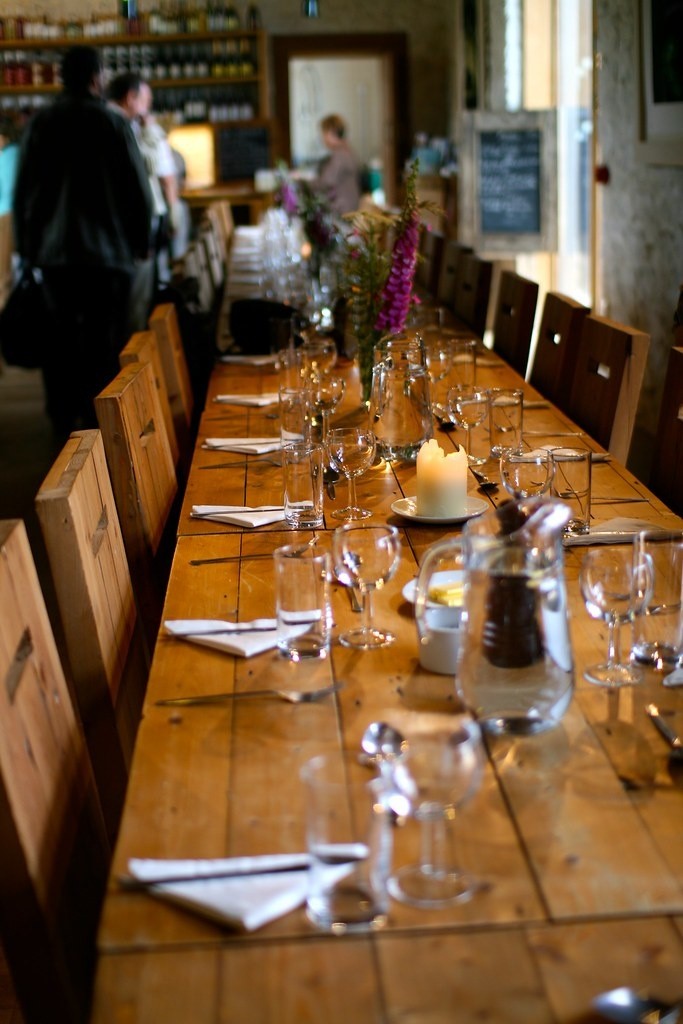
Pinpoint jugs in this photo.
[414,497,575,737]
[367,331,435,466]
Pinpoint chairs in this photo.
[1,197,683,1023]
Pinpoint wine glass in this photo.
[446,385,490,466]
[420,338,451,418]
[263,208,345,464]
[578,548,655,688]
[332,522,400,650]
[376,712,485,912]
[325,428,377,521]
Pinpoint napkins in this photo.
[221,356,277,364]
[212,392,298,407]
[162,613,321,658]
[116,841,374,935]
[187,499,311,530]
[199,436,298,456]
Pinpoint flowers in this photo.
[343,159,447,334]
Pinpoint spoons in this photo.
[334,565,362,612]
[361,721,407,828]
[323,466,340,500]
[472,469,499,489]
[311,415,323,443]
[435,414,454,429]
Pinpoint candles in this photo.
[416,438,469,517]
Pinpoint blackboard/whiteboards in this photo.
[191,120,278,227]
[463,109,558,254]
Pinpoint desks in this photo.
[179,179,282,227]
[94,222,680,1022]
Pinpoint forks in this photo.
[189,536,320,566]
[530,480,650,502]
[199,455,281,470]
[154,681,344,708]
[483,426,582,436]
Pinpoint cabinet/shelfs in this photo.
[0,23,270,132]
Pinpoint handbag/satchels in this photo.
[0,258,76,370]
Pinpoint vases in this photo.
[357,333,385,398]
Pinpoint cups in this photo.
[486,388,524,460]
[499,446,555,500]
[273,543,331,665]
[446,339,478,392]
[281,441,323,530]
[548,447,593,538]
[296,749,392,937]
[416,606,465,675]
[630,527,683,669]
[280,387,314,455]
[277,349,303,414]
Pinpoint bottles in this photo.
[0,4,256,127]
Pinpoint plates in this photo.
[390,496,490,524]
[402,570,465,609]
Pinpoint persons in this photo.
[13,41,153,459]
[0,73,192,298]
[305,116,374,229]
